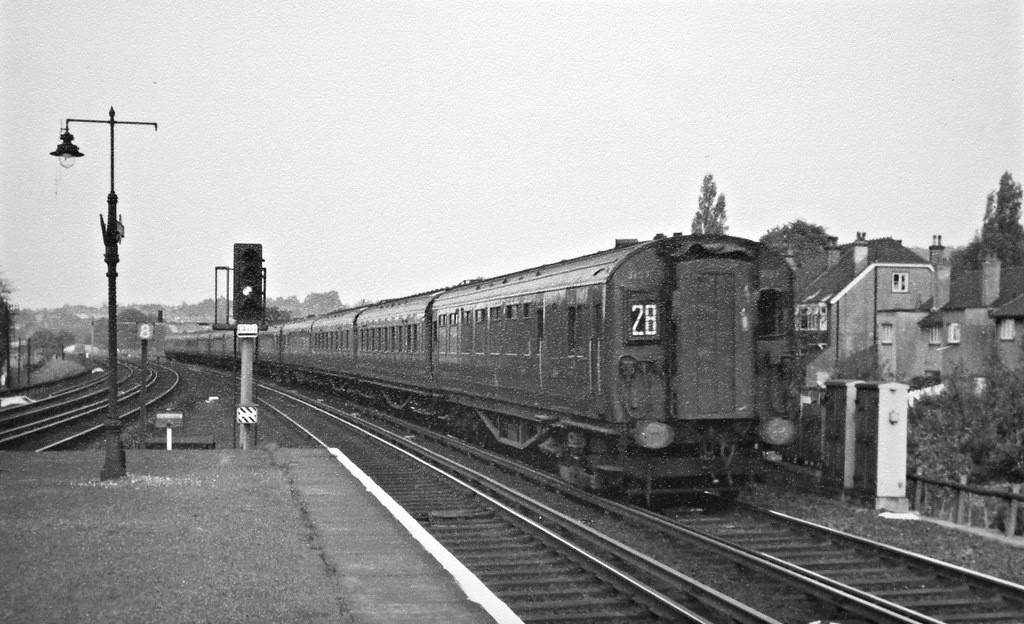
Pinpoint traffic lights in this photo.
[158,310,163,322]
[233,243,262,320]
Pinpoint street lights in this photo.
[50,105,157,481]
[91,321,97,369]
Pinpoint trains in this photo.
[165,233,800,505]
